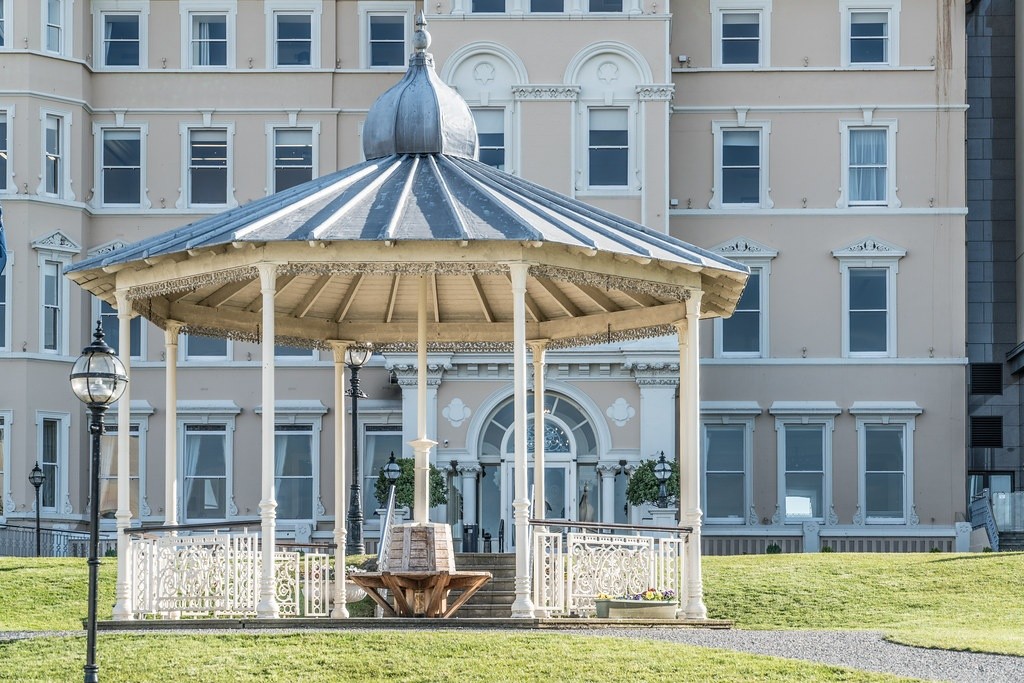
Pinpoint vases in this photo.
[594,598,611,618]
[609,599,680,618]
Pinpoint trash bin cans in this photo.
[462,521,478,553]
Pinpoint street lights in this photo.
[69,320,128,683]
[29,461,46,556]
[343,348,372,570]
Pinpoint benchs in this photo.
[349,570,493,619]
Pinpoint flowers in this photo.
[615,585,675,601]
[595,593,608,599]
